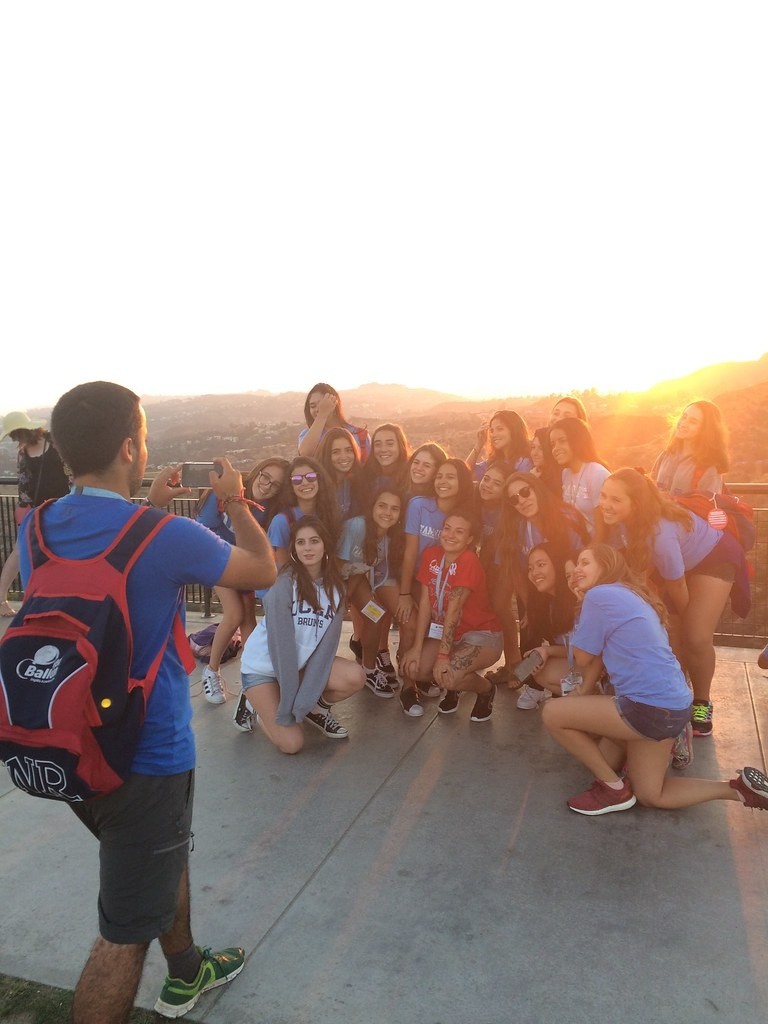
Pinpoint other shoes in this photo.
[507,672,525,689]
[350,633,362,658]
[485,666,517,685]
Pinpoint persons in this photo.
[192,383,767,816]
[17,378,279,1023]
[0,410,71,618]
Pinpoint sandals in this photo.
[0,600,17,617]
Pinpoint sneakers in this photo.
[672,721,693,769]
[362,667,395,698]
[232,688,257,732]
[730,767,768,811]
[437,689,464,713]
[566,780,636,815]
[305,711,349,738]
[470,677,497,721]
[202,666,226,705]
[415,681,440,698]
[375,649,395,678]
[516,685,543,709]
[156,942,246,1019]
[399,686,423,717]
[691,699,714,736]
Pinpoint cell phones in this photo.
[180,462,224,490]
[513,649,544,684]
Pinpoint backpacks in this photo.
[665,490,757,551]
[187,622,242,664]
[0,498,196,808]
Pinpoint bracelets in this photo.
[217,486,266,516]
[400,592,412,596]
[141,496,156,508]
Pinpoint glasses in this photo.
[259,470,281,494]
[290,472,317,485]
[509,486,532,505]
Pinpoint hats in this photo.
[0,412,46,440]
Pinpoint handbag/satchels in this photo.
[14,504,34,525]
